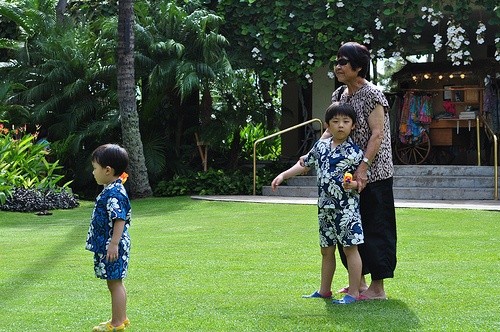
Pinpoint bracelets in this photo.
[363,157,371,166]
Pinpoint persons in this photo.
[299,42,397,300]
[271,101,371,303]
[84,143,131,332]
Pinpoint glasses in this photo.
[334,58,351,66]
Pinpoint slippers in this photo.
[302,291,334,299]
[357,294,386,301]
[333,294,357,305]
[339,287,369,293]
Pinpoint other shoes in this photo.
[98,318,131,326]
[93,321,126,332]
